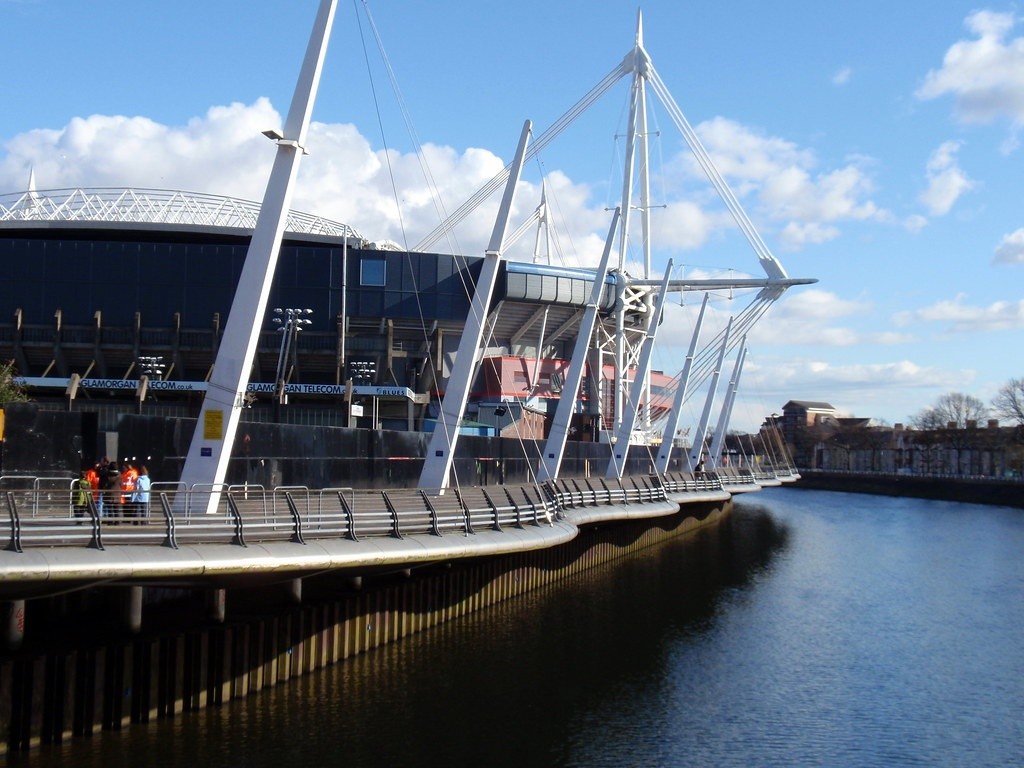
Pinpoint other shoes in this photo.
[77,522,84,525]
[108,521,113,526]
[114,521,120,525]
[133,521,138,525]
[122,520,131,523]
[102,521,108,524]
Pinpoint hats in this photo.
[109,461,118,470]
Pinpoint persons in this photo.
[73,457,152,525]
